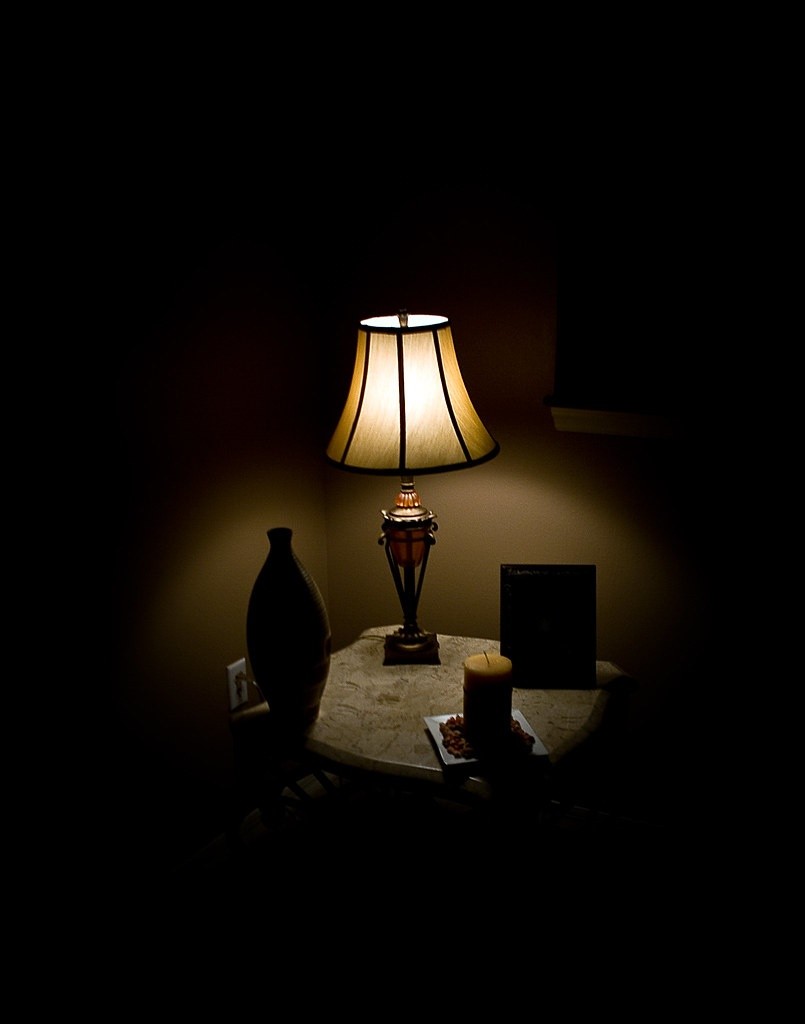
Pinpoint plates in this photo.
[422,708,549,765]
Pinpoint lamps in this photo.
[322,313,502,659]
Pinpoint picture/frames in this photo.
[501,564,600,690]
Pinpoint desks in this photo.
[234,633,631,957]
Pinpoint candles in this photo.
[462,651,513,744]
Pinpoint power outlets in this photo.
[227,658,248,710]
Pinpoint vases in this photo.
[246,527,333,725]
